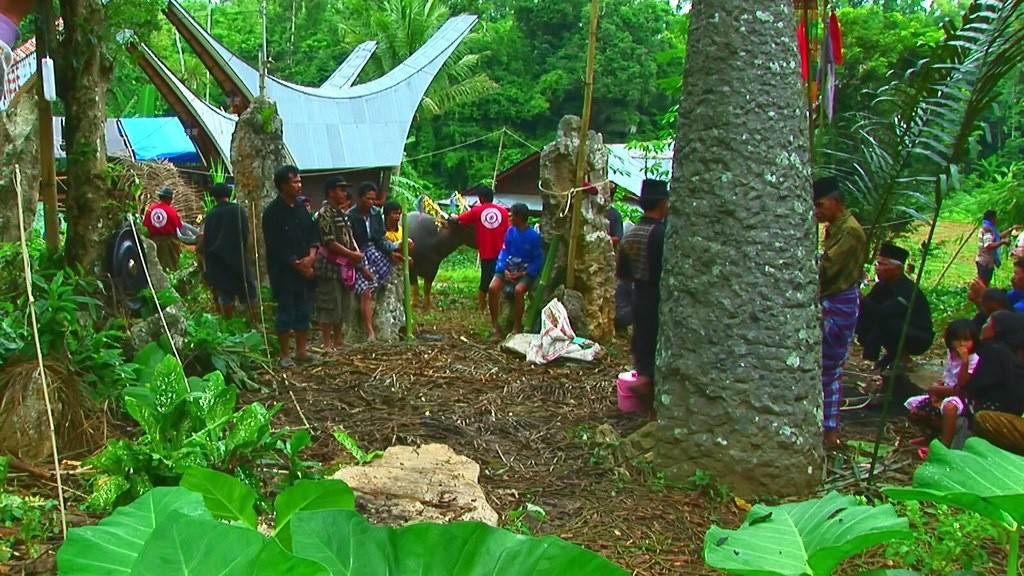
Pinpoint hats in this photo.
[641,179,667,201]
[212,183,229,197]
[325,176,352,187]
[814,176,840,199]
[879,244,910,264]
[158,187,173,198]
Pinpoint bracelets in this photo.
[1008,228,1012,233]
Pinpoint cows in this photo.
[380,211,478,310]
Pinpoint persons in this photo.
[488,204,546,343]
[976,207,1024,286]
[141,165,416,368]
[857,243,937,378]
[909,258,1024,457]
[615,179,670,375]
[813,176,868,453]
[1009,224,1024,260]
[607,180,623,245]
[453,186,510,318]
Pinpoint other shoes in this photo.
[909,436,929,444]
[866,356,919,377]
[918,447,930,459]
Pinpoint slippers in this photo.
[279,359,296,368]
[305,354,321,362]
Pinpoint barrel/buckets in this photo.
[616,369,653,414]
